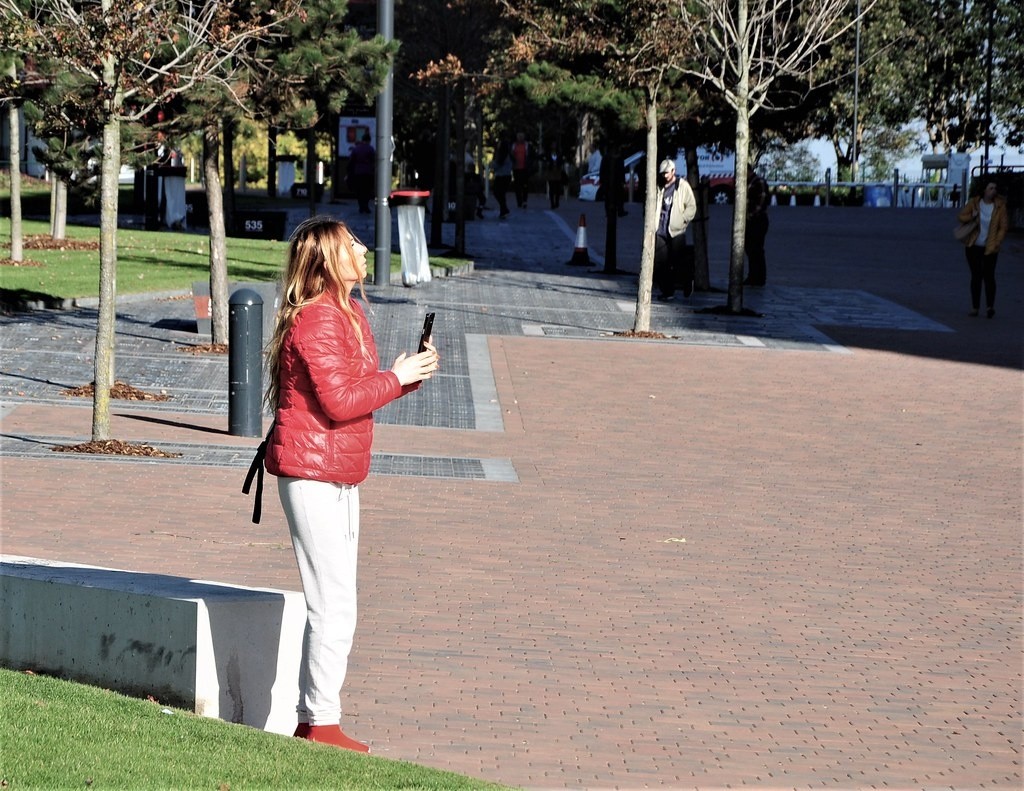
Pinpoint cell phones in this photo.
[417,312,435,354]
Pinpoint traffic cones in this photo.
[566,214,596,267]
[770,189,777,206]
[788,189,796,207]
[813,188,822,207]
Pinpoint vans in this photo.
[578,146,736,205]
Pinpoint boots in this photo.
[476,208,484,219]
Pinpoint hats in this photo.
[659,159,675,173]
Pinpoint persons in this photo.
[744,162,771,287]
[242,213,441,756]
[654,159,697,302]
[462,131,569,220]
[345,133,378,214]
[587,140,627,219]
[957,182,1008,318]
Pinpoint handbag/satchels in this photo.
[952,198,978,243]
[495,161,512,176]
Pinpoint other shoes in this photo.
[684,280,694,298]
[743,275,767,289]
[657,293,674,300]
[293,723,310,738]
[358,205,371,214]
[517,202,528,209]
[307,726,370,754]
[986,309,995,318]
[968,310,978,317]
[498,208,510,221]
